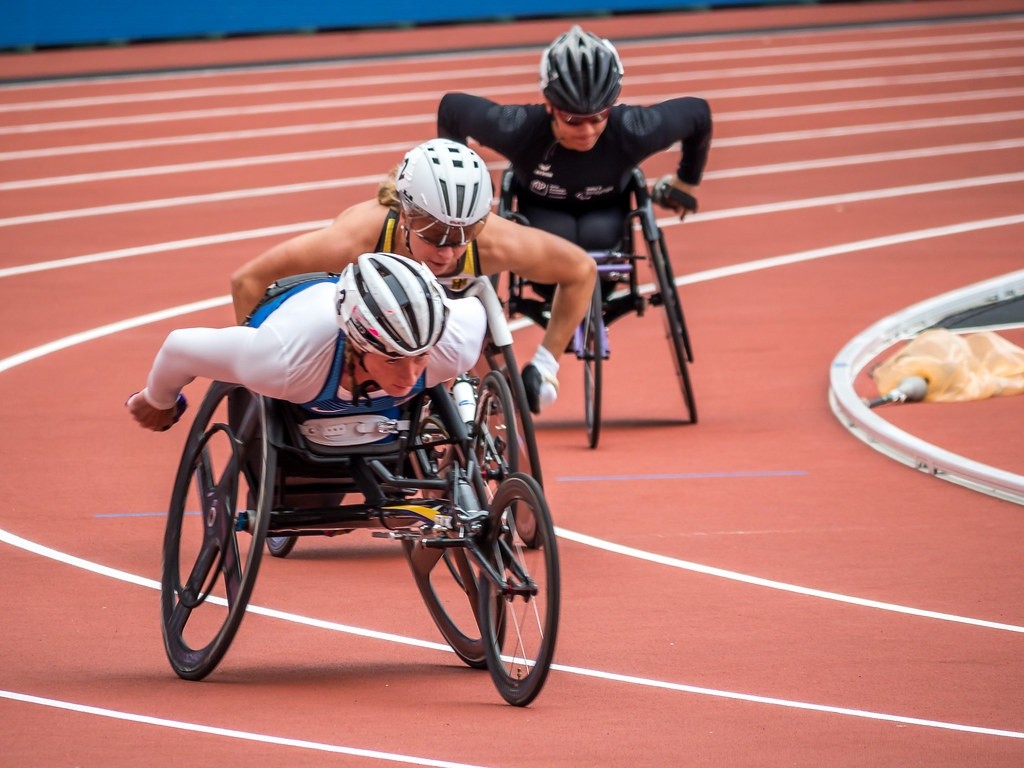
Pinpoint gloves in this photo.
[524,343,560,415]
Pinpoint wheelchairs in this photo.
[156,262,568,711]
[485,161,698,449]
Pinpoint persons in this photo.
[437,26,712,305]
[231,137,598,413]
[127,250,488,431]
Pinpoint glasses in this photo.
[554,109,616,124]
[404,204,490,246]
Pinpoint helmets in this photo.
[334,252,451,361]
[397,137,494,227]
[539,30,626,116]
